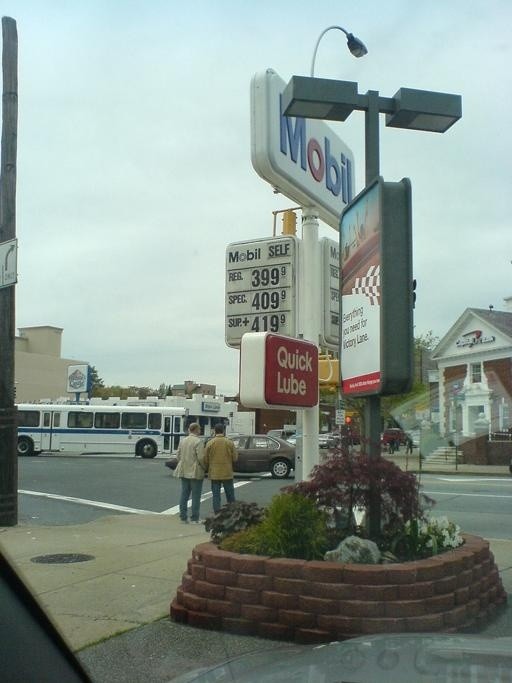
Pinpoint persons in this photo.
[405,437,413,454]
[172,423,239,525]
[389,437,400,454]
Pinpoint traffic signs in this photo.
[0,238,18,286]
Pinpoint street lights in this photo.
[279,72,463,544]
[308,22,368,79]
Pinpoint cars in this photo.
[166,428,406,479]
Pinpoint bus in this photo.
[15,402,187,458]
